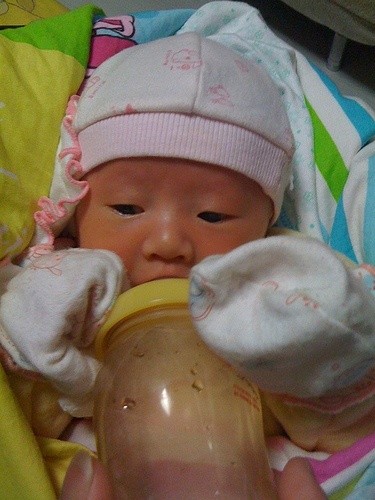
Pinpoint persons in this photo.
[0,33,375,500]
[54,446,330,500]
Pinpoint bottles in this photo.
[95,278,279,500]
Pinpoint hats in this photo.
[71,31,295,229]
